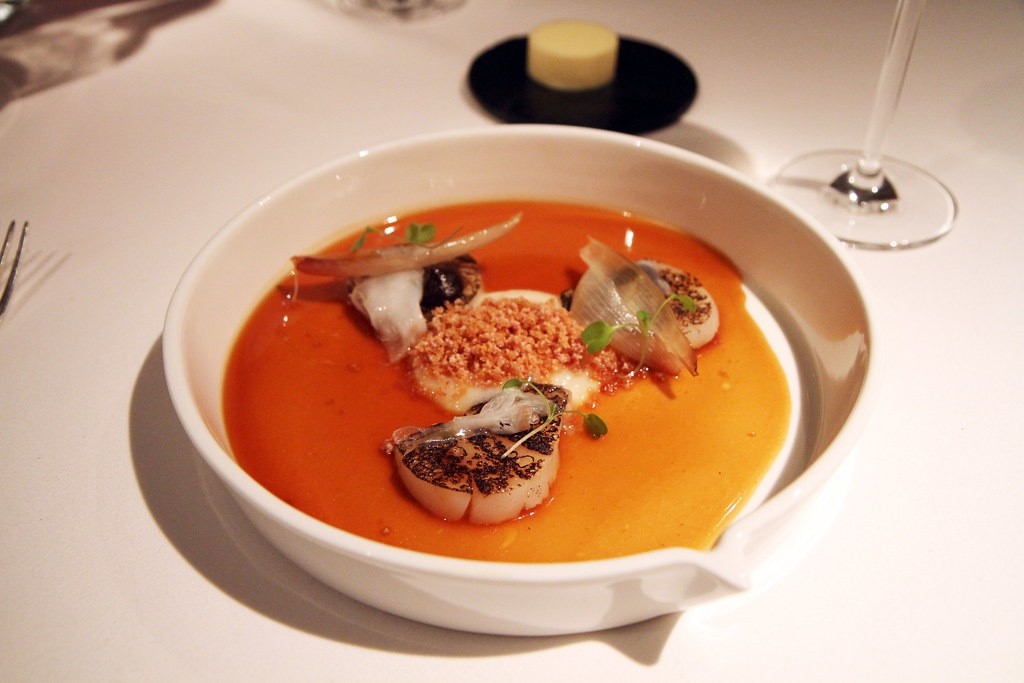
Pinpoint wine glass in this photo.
[775,1,960,245]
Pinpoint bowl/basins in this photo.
[157,124,874,639]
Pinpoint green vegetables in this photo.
[352,221,697,464]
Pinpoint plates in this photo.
[466,33,697,139]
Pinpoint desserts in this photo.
[526,21,619,91]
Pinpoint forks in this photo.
[0,219,29,319]
